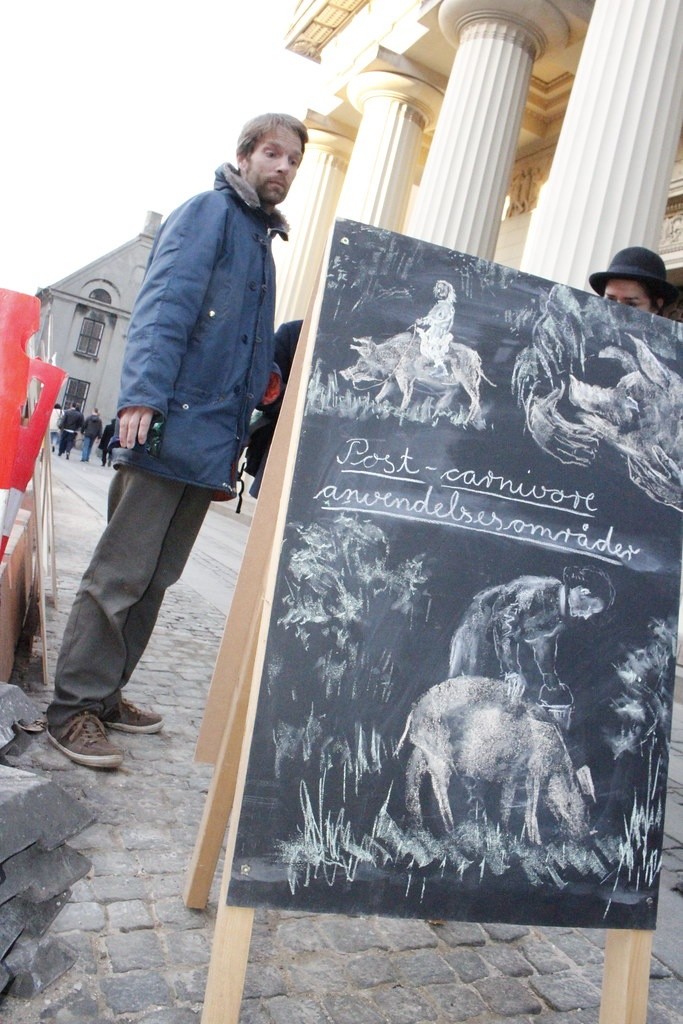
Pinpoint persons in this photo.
[245,320,304,498]
[588,246,680,317]
[58,402,84,460]
[50,403,64,452]
[46,114,309,767]
[97,419,116,467]
[81,408,103,463]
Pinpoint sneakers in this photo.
[46,710,127,772]
[99,696,166,733]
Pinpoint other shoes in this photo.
[101,463,105,466]
[66,453,69,459]
[79,458,89,463]
[58,452,62,456]
[108,460,112,467]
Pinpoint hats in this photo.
[587,245,680,308]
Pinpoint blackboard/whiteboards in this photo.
[215,215,683,930]
